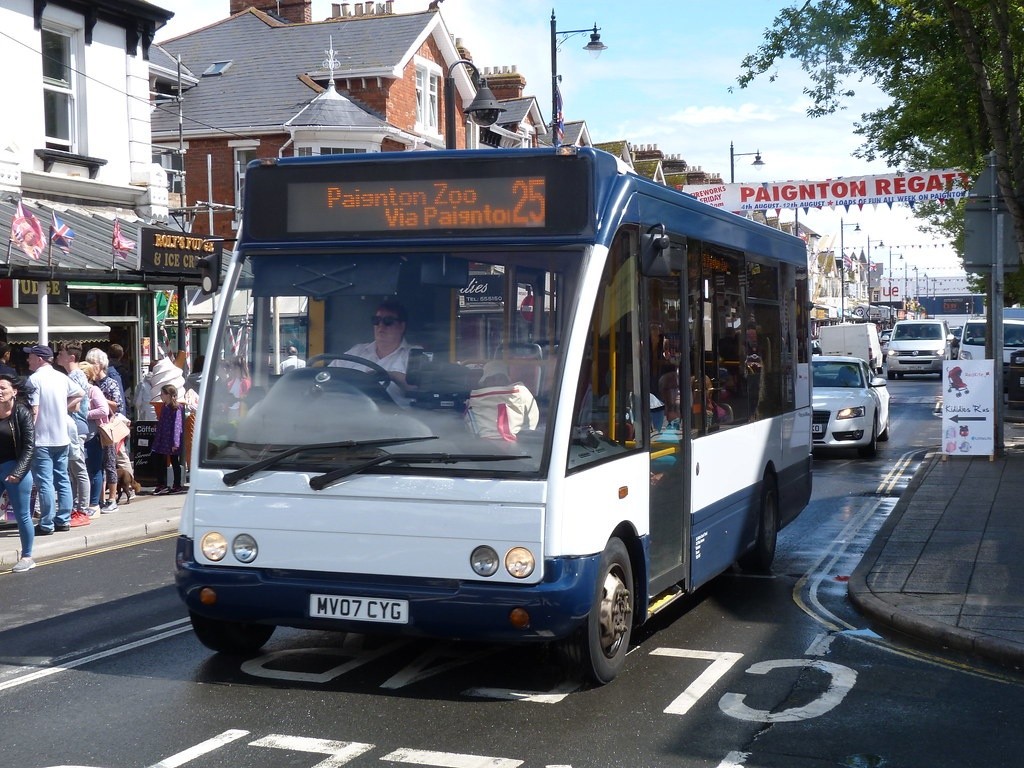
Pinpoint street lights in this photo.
[730,141,766,187]
[868,234,886,323]
[890,250,905,329]
[444,59,507,151]
[905,262,918,320]
[551,8,607,147]
[916,271,938,315]
[840,217,862,324]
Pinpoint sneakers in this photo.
[100,499,119,514]
[86,504,101,519]
[152,484,169,495]
[69,511,91,527]
[12,558,37,573]
[166,484,183,495]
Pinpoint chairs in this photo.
[717,403,733,422]
[494,342,542,396]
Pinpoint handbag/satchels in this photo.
[97,414,130,448]
[463,381,540,442]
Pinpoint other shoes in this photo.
[33,524,54,536]
[54,523,70,531]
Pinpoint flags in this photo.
[8,198,46,262]
[49,209,76,256]
[111,217,136,260]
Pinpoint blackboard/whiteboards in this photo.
[133,420,159,487]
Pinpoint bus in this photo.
[175,145,816,686]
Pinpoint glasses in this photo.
[371,315,404,326]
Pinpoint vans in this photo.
[886,319,954,379]
[951,320,1024,364]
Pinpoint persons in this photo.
[23,345,86,535]
[0,374,37,572]
[0,341,251,520]
[152,385,183,495]
[328,299,426,406]
[596,302,763,544]
[274,346,307,375]
[462,360,539,450]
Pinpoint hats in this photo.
[22,345,55,364]
[477,359,513,385]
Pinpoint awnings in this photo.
[0,302,111,346]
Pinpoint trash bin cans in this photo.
[1008,348,1024,409]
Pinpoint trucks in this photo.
[818,322,884,376]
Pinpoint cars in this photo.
[878,327,963,361]
[811,356,891,457]
